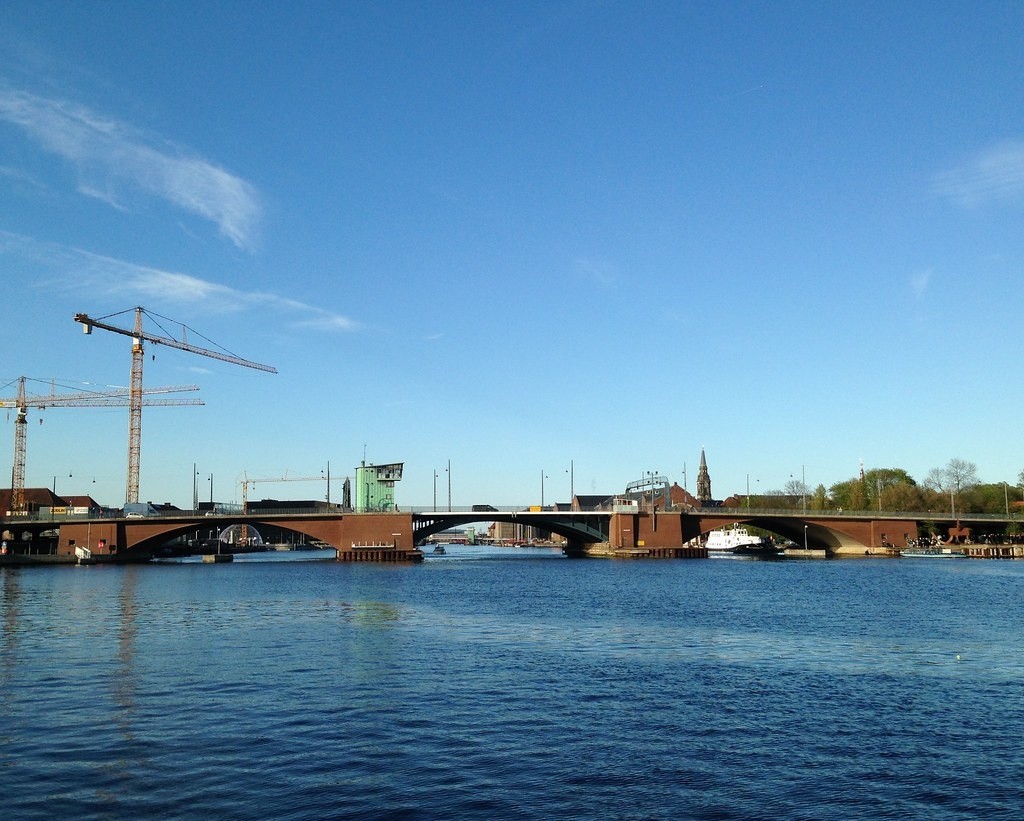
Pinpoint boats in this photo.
[704,523,765,551]
[900,553,967,557]
[732,534,783,554]
[960,546,1014,558]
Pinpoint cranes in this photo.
[0,374,205,510]
[72,304,278,505]
[235,472,355,547]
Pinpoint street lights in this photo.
[804,525,808,550]
